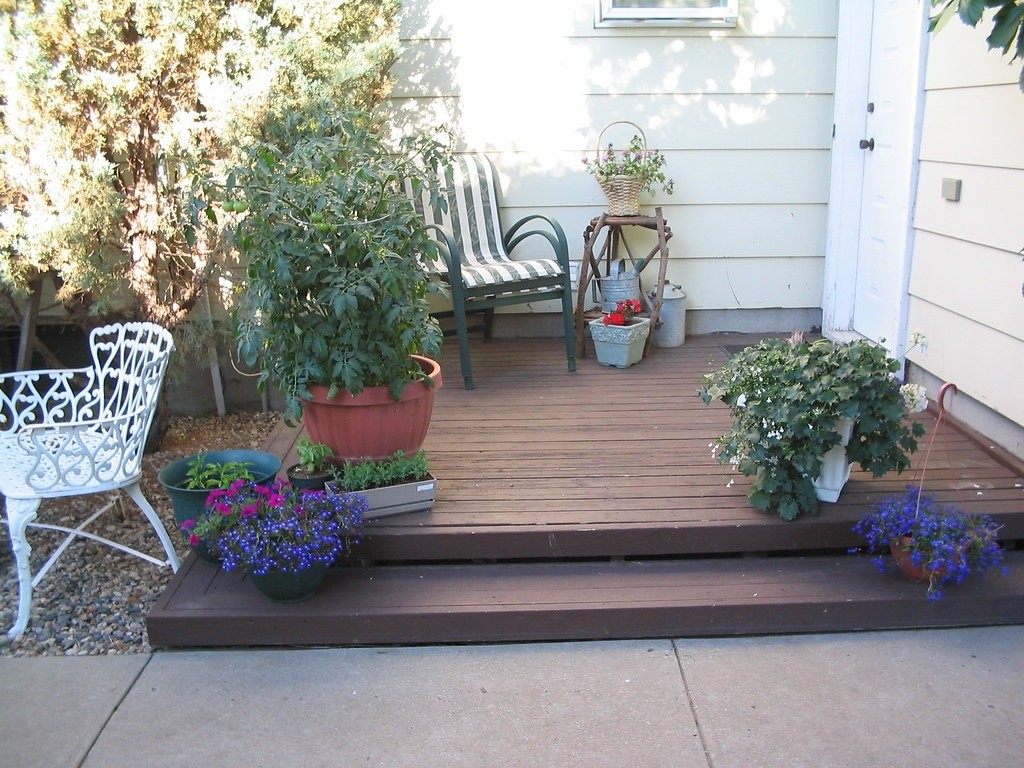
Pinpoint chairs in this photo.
[399,151,576,390]
[0,324,181,641]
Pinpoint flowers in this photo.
[178,477,322,550]
[848,483,1008,607]
[208,493,368,576]
[697,327,932,522]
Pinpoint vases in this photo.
[254,561,334,598]
[890,533,972,585]
[769,403,860,502]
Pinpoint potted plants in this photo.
[288,440,339,501]
[581,120,675,216]
[325,448,437,521]
[588,299,652,369]
[157,450,282,533]
[177,101,456,464]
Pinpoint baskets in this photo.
[595,121,649,216]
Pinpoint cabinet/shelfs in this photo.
[575,207,673,359]
